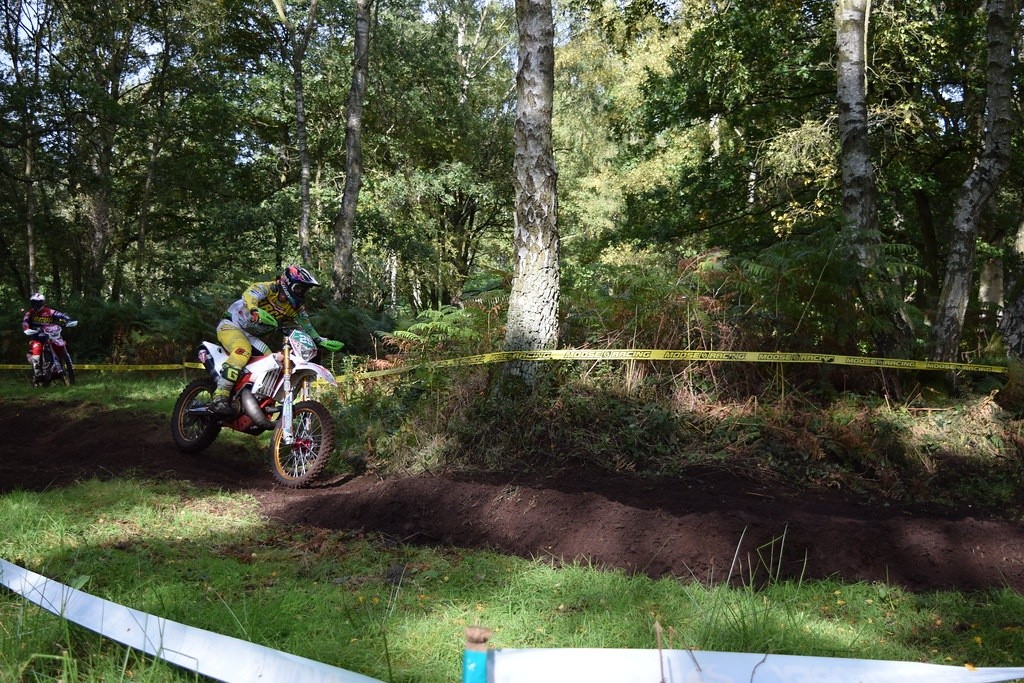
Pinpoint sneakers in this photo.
[69,372,75,384]
[207,399,235,417]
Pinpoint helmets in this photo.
[30,292,46,301]
[276,263,320,310]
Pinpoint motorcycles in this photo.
[172,321,338,489]
[23,323,78,386]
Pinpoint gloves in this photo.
[250,308,259,324]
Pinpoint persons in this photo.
[23,293,70,376]
[212,266,344,415]
